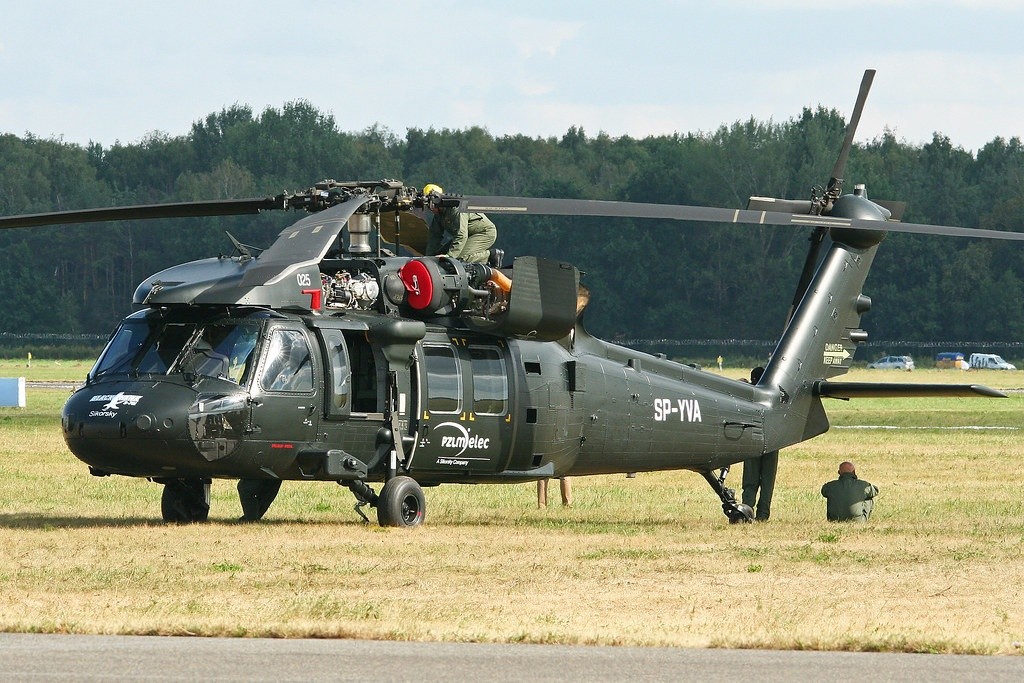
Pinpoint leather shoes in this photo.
[491,249,504,270]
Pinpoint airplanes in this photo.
[1,67,1024,541]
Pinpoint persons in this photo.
[741,365,778,521]
[421,183,505,270]
[821,462,879,523]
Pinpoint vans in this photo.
[865,356,916,371]
[969,352,1017,370]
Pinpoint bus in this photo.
[936,353,965,360]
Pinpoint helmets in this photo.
[424,185,442,197]
